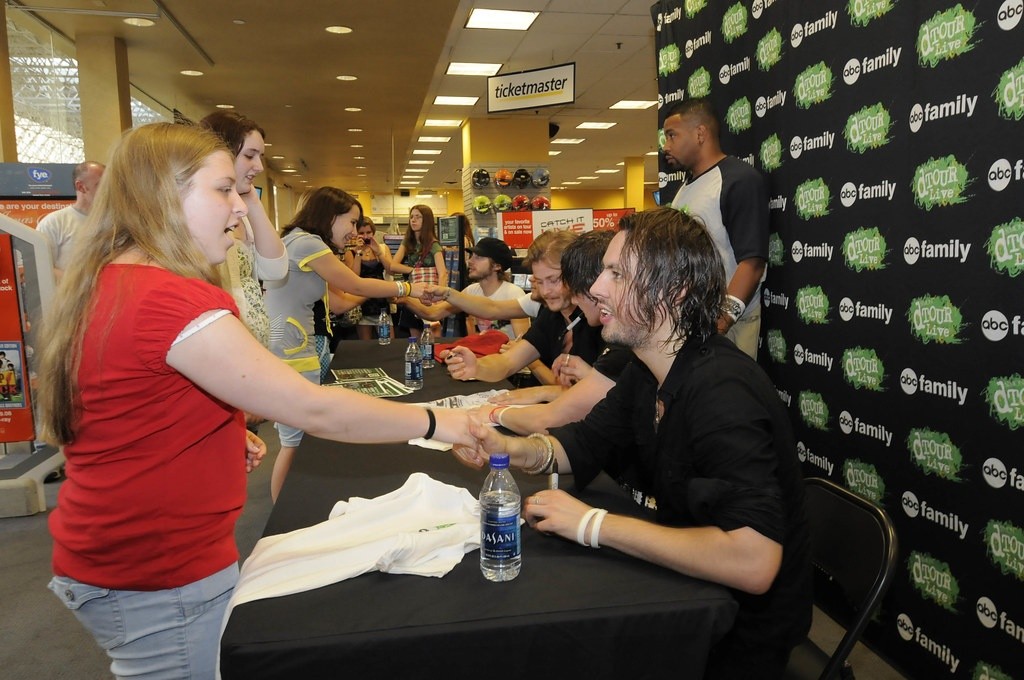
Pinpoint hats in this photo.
[465,237,516,273]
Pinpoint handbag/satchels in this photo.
[409,239,448,301]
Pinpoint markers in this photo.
[447,353,459,359]
[549,458,559,490]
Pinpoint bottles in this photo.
[420,323,436,368]
[378,308,390,345]
[478,452,522,583]
[404,336,424,389]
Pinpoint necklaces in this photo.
[363,246,371,256]
[654,385,661,426]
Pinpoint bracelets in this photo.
[720,291,748,323]
[404,280,412,297]
[488,406,502,424]
[396,281,403,297]
[523,432,554,477]
[443,286,451,301]
[423,407,436,440]
[576,506,607,547]
[499,407,516,428]
[355,249,362,256]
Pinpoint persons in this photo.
[660,100,771,360]
[461,207,816,680]
[263,186,448,505]
[405,229,633,440]
[35,166,103,482]
[44,122,488,680]
[196,111,290,435]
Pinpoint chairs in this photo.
[791,477,901,680]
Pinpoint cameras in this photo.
[364,238,370,244]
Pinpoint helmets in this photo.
[531,195,551,211]
[494,169,514,189]
[531,168,550,188]
[471,168,491,190]
[472,195,492,216]
[513,168,532,190]
[493,194,512,213]
[512,194,531,212]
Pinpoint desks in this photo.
[219,337,740,680]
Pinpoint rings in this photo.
[535,495,542,505]
[565,354,570,367]
